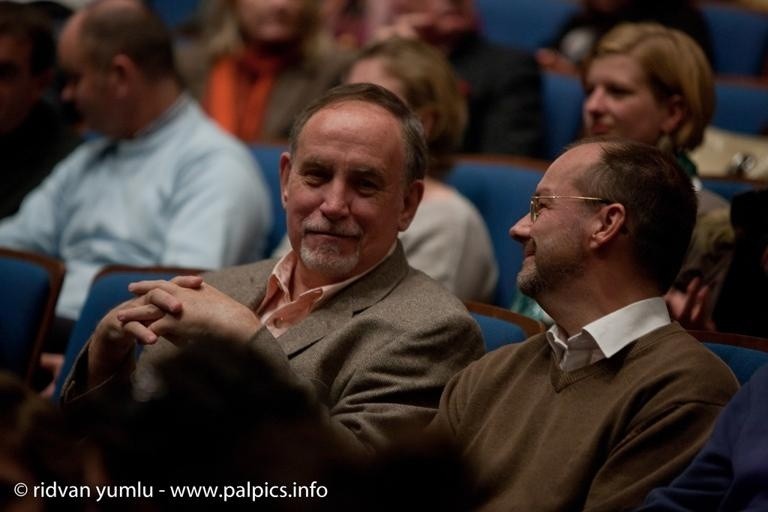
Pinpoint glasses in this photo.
[530,193,603,223]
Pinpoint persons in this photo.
[0,0,768,399]
[637,363,768,512]
[424,135,741,512]
[59,82,486,512]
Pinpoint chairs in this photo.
[3,7,768,400]
